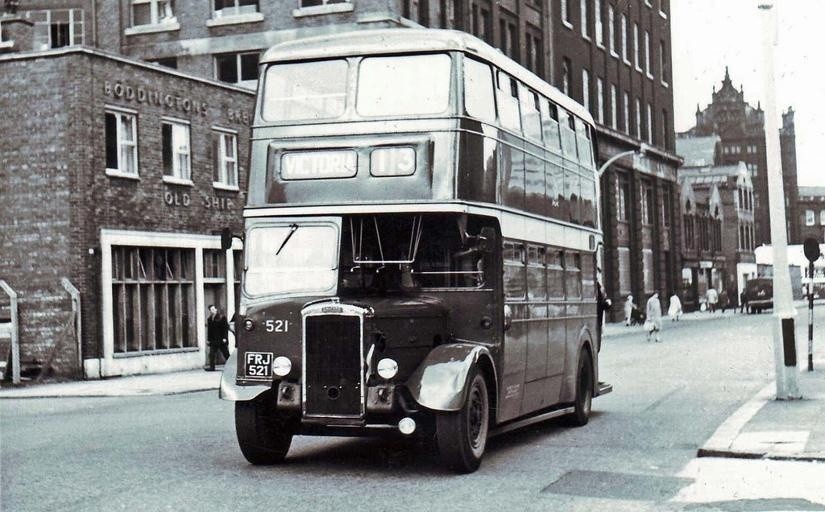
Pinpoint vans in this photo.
[743,277,774,314]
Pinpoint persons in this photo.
[624,295,637,328]
[595,266,614,349]
[668,292,683,322]
[801,282,809,305]
[643,290,662,343]
[204,304,231,371]
[700,283,767,316]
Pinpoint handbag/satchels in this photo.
[643,317,655,332]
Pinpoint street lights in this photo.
[752,3,803,397]
[596,141,653,324]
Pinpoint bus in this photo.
[217,30,613,473]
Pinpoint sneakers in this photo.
[645,334,663,343]
